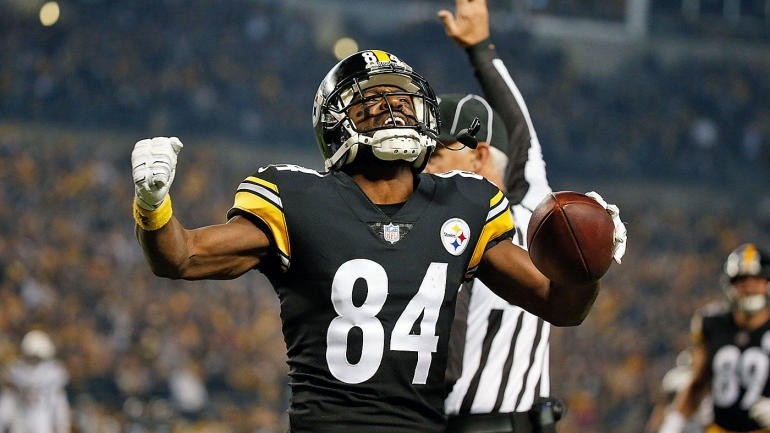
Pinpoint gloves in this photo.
[127,132,184,211]
[584,187,629,264]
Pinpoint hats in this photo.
[431,90,492,150]
[721,243,770,313]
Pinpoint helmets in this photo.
[312,47,440,173]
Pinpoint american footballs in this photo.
[525,189,616,294]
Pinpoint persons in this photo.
[419,0,565,432]
[131,48,629,432]
[3,145,770,433]
